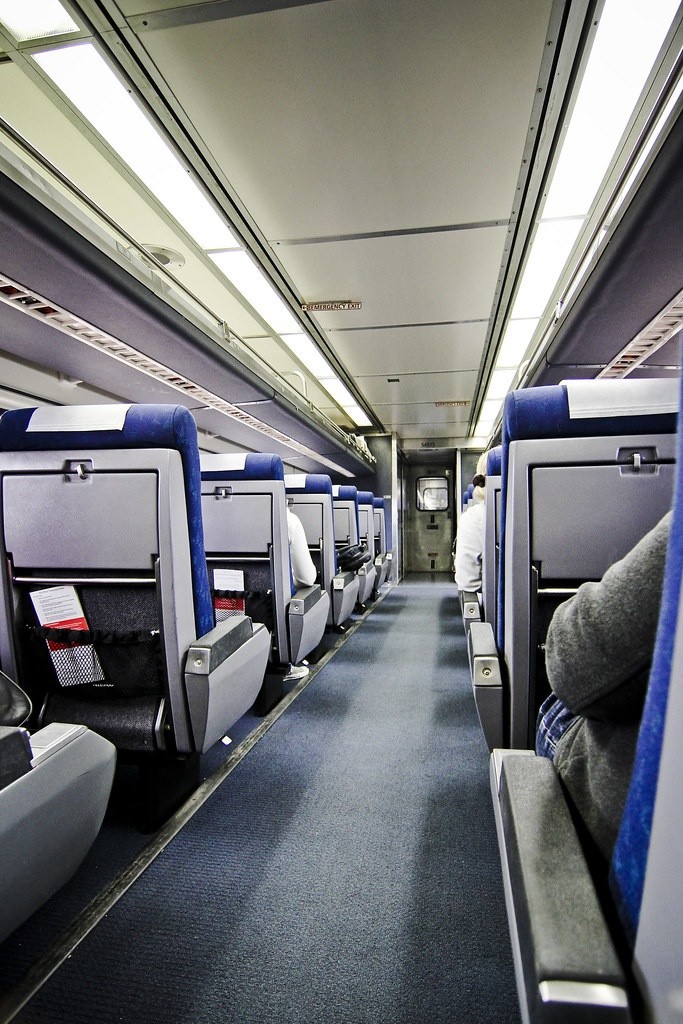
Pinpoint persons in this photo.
[285,499,316,592]
[535,511,673,863]
[453,447,487,604]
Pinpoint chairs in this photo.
[0,401,388,949]
[453,374,683,1024]
